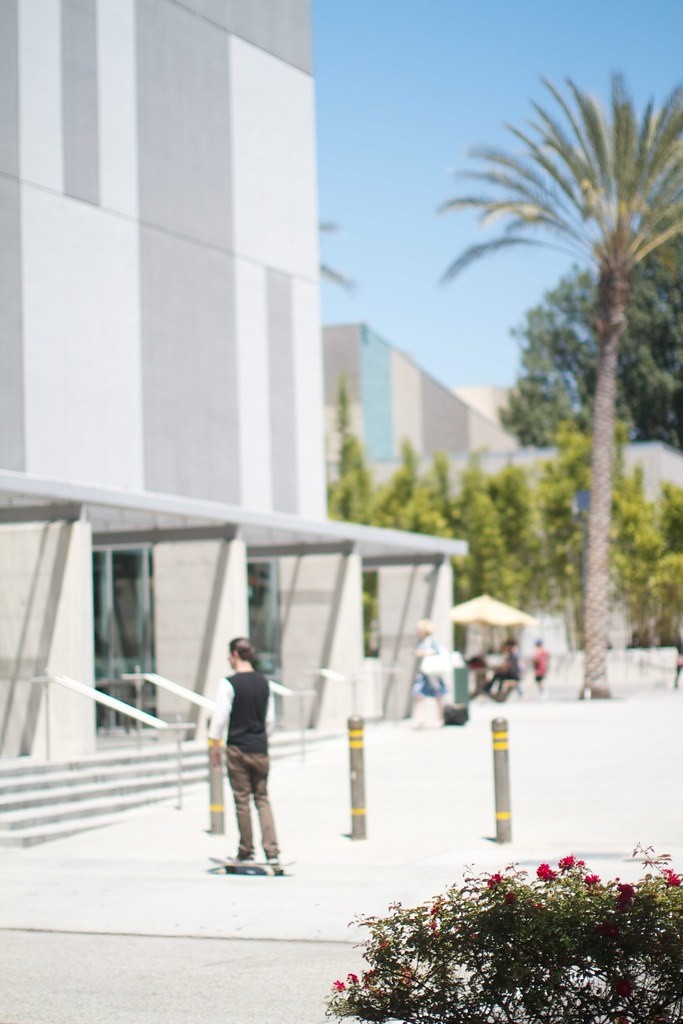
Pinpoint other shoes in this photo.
[266,858,279,864]
[233,852,252,862]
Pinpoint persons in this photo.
[482,627,522,698]
[531,640,550,695]
[674,630,683,689]
[209,638,281,866]
[406,620,448,730]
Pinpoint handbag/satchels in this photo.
[420,638,447,676]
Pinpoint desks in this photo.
[471,665,507,702]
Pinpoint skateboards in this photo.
[208,854,289,877]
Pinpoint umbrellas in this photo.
[441,594,536,650]
